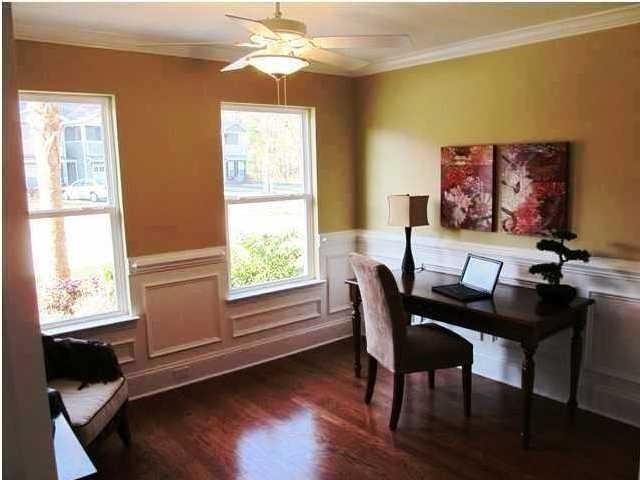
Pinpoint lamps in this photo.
[387,193,429,273]
[244,53,310,84]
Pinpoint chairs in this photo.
[346,251,472,433]
[43,329,131,472]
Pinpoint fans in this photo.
[135,2,411,106]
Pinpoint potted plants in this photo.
[531,226,591,304]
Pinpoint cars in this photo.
[60,178,107,202]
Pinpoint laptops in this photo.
[433,254,504,303]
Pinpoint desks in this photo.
[342,268,595,452]
